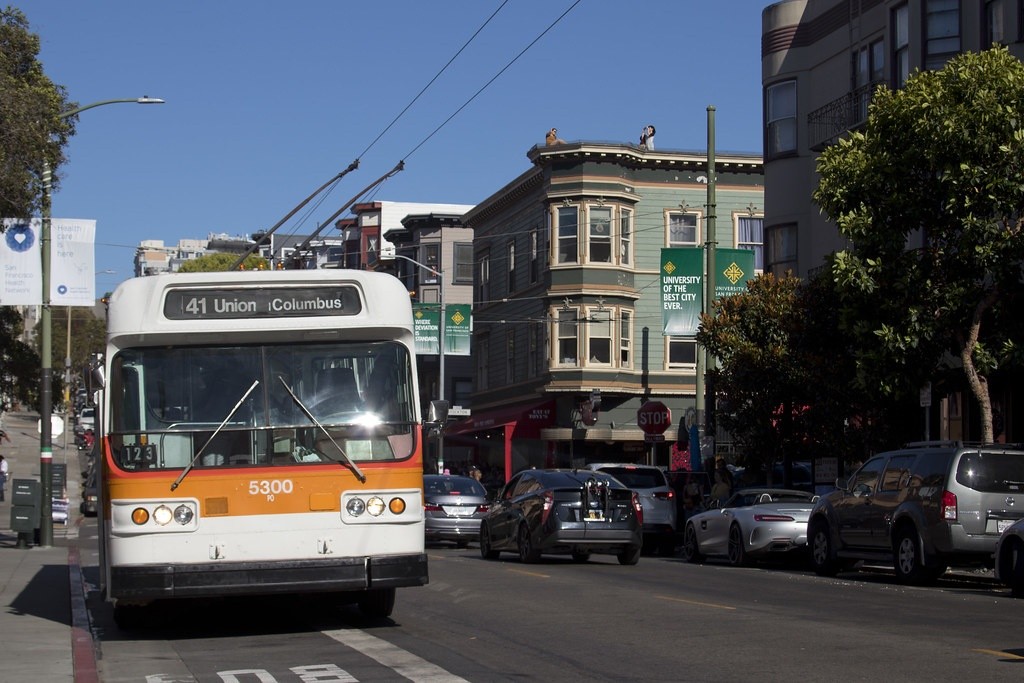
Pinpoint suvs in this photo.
[806,439,1023,588]
[583,462,678,557]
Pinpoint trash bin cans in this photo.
[52,462,71,526]
[9,477,42,549]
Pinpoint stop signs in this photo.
[637,401,672,435]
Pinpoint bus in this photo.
[89,266,451,630]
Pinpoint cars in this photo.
[423,472,494,543]
[683,488,823,567]
[994,517,1024,590]
[75,386,95,436]
[479,467,645,565]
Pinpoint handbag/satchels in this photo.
[684,498,693,509]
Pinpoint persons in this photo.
[470,469,482,482]
[545,128,568,145]
[640,125,656,151]
[0,455,8,501]
[682,458,736,515]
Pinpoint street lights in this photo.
[30,98,165,549]
[379,254,447,473]
[62,270,117,454]
